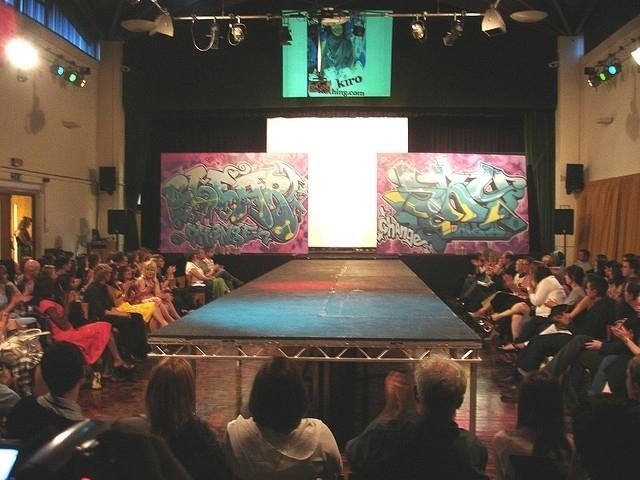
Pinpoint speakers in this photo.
[553,209,574,235]
[108,209,130,234]
[565,164,584,190]
[99,167,116,191]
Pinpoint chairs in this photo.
[176,275,211,305]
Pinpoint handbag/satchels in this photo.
[0,329,50,366]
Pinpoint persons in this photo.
[143,355,238,480]
[1,246,245,399]
[5,341,88,441]
[222,355,343,480]
[321,22,355,77]
[493,370,577,480]
[454,247,639,399]
[345,354,488,480]
[570,394,639,480]
[17,217,34,265]
[1,383,22,429]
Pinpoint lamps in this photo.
[48,50,91,87]
[171,0,484,52]
[584,37,640,87]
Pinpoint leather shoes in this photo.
[496,343,524,403]
